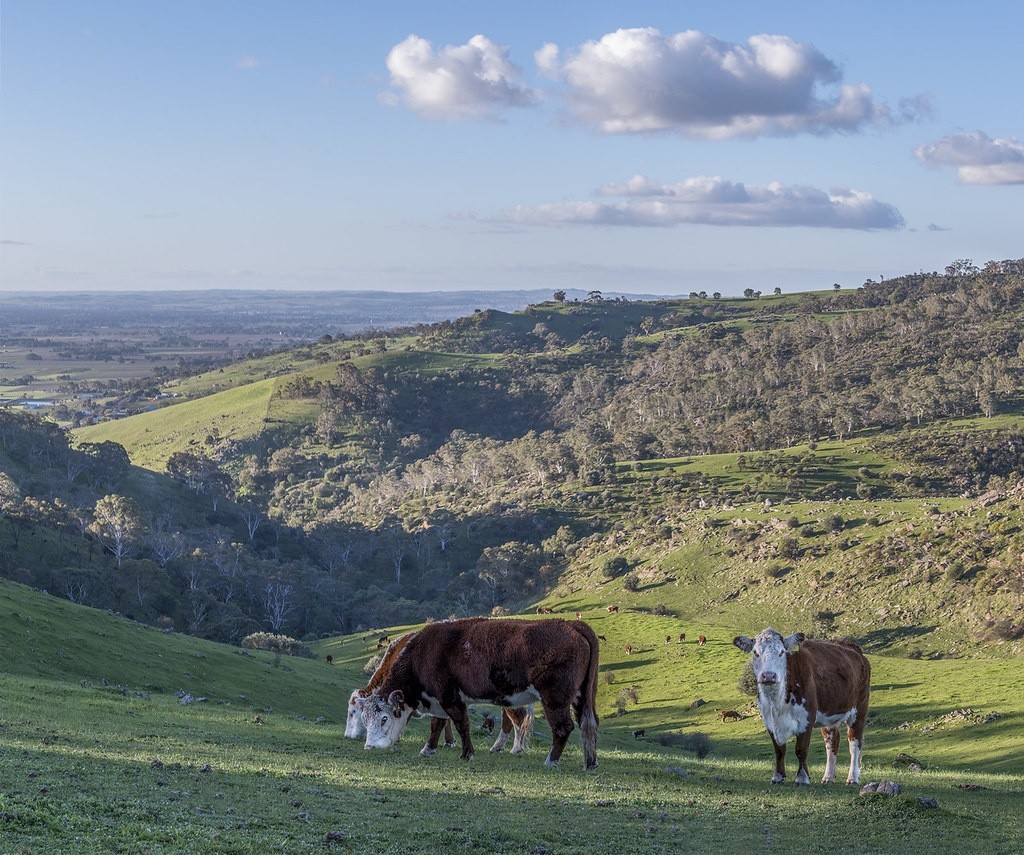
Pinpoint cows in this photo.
[665,635,671,643]
[544,608,553,615]
[678,633,685,642]
[343,617,607,771]
[721,710,744,723]
[576,613,582,620]
[624,644,631,655]
[733,628,872,786]
[536,608,541,615]
[632,729,645,739]
[698,635,706,645]
[326,655,333,663]
[607,605,618,612]
